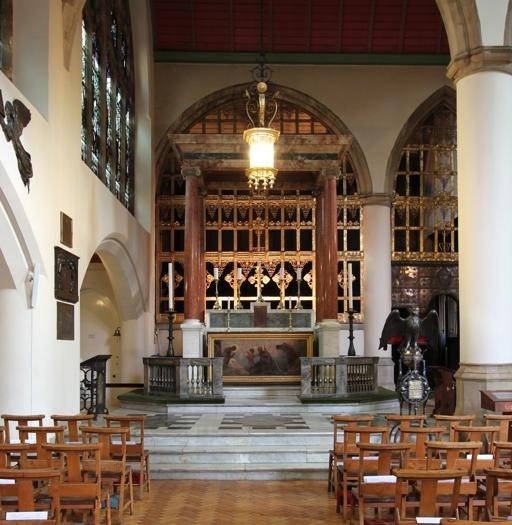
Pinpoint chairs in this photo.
[0,412,150,525]
[326,413,512,525]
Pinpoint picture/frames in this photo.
[60,212,74,248]
[208,334,313,384]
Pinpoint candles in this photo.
[214,268,218,279]
[237,268,242,280]
[168,263,175,311]
[279,268,284,278]
[297,269,301,279]
[348,263,353,311]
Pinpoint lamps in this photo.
[242,0,281,190]
